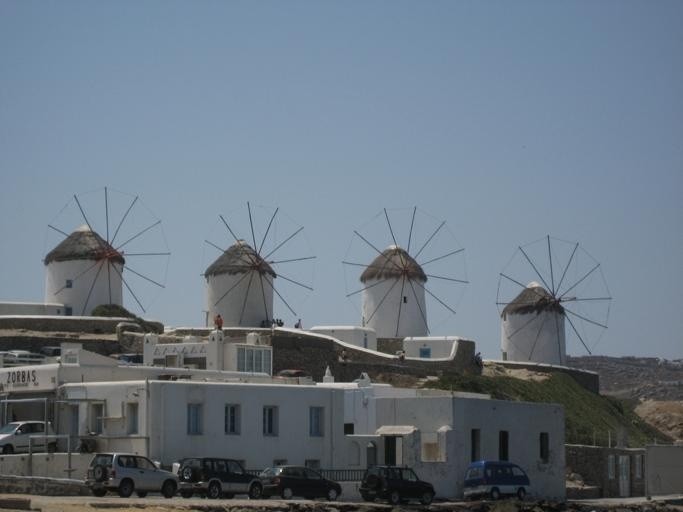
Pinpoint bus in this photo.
[109,353,142,364]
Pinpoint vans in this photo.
[461,460,530,500]
[38,346,61,358]
[3,349,30,367]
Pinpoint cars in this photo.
[258,465,341,501]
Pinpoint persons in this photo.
[260,319,284,328]
[341,348,348,362]
[400,350,406,356]
[214,314,223,330]
[298,319,302,328]
[475,352,484,369]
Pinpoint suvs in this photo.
[176,456,263,500]
[85,452,179,500]
[0,421,58,456]
[357,465,435,506]
